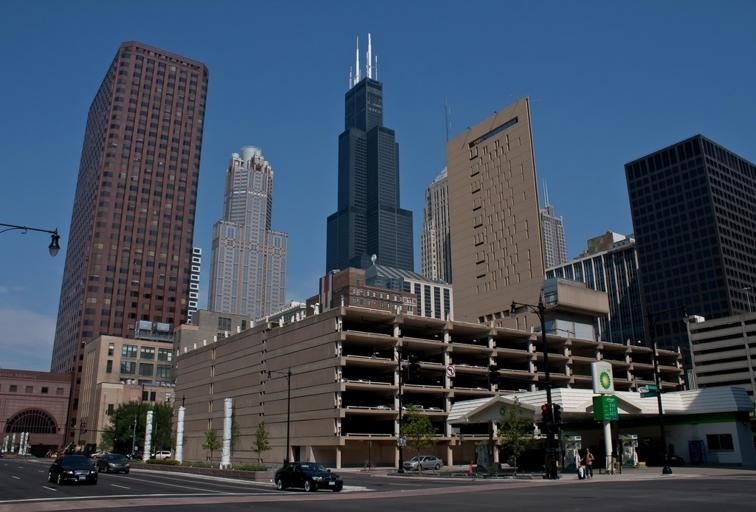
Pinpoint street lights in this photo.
[742,286,751,311]
[649,313,705,474]
[265,367,291,467]
[510,295,559,479]
[372,342,404,472]
[0,222,60,257]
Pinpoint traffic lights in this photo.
[540,404,550,423]
[553,403,561,424]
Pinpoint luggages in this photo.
[577,461,586,479]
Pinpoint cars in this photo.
[403,455,443,471]
[44,441,170,486]
[273,462,343,494]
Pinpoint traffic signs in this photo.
[640,391,659,398]
[637,387,658,393]
[646,385,657,390]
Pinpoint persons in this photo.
[575,447,596,479]
